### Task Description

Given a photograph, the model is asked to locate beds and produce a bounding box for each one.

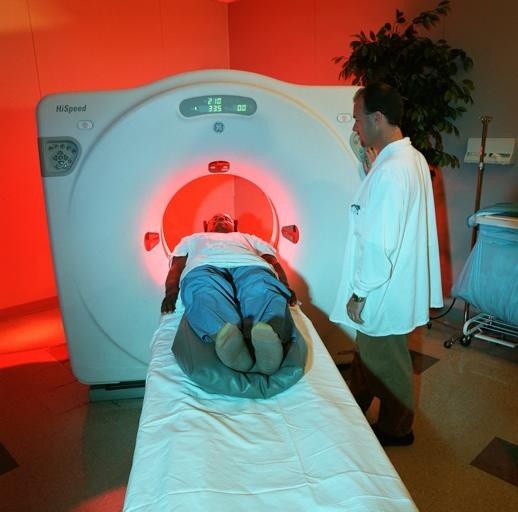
[123,276,417,512]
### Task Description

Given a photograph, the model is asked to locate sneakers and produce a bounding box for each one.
[371,423,414,446]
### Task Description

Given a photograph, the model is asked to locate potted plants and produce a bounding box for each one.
[329,2,477,181]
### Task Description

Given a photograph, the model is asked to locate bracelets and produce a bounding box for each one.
[352,292,366,303]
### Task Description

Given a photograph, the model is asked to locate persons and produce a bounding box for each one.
[328,84,444,447]
[161,212,297,377]
